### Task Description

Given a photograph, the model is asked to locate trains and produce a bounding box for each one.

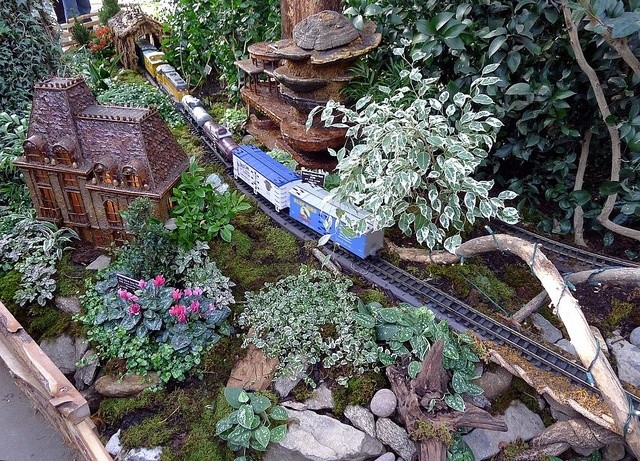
[135,41,384,259]
